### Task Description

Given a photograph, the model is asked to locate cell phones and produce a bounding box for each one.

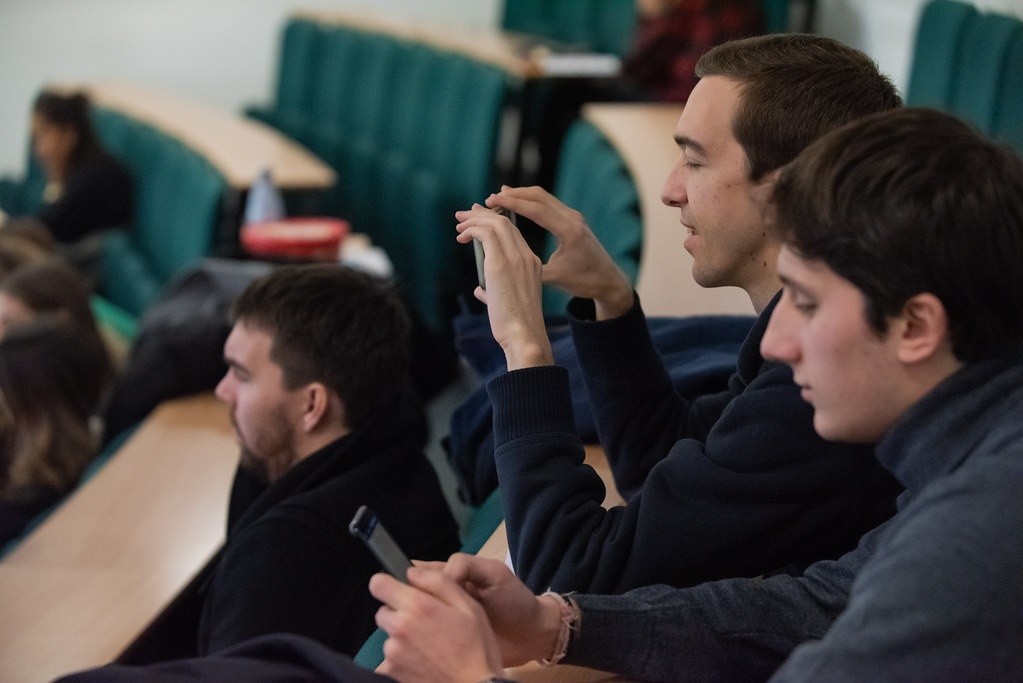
[473,207,516,290]
[349,504,412,586]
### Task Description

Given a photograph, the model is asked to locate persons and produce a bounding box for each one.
[189,260,467,659]
[343,108,1022,682]
[455,32,908,599]
[0,88,133,541]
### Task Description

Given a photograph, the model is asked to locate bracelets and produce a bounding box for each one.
[547,592,579,665]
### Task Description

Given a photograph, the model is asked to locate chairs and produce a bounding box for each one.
[263,3,647,658]
[17,98,234,331]
[904,0,1023,163]
